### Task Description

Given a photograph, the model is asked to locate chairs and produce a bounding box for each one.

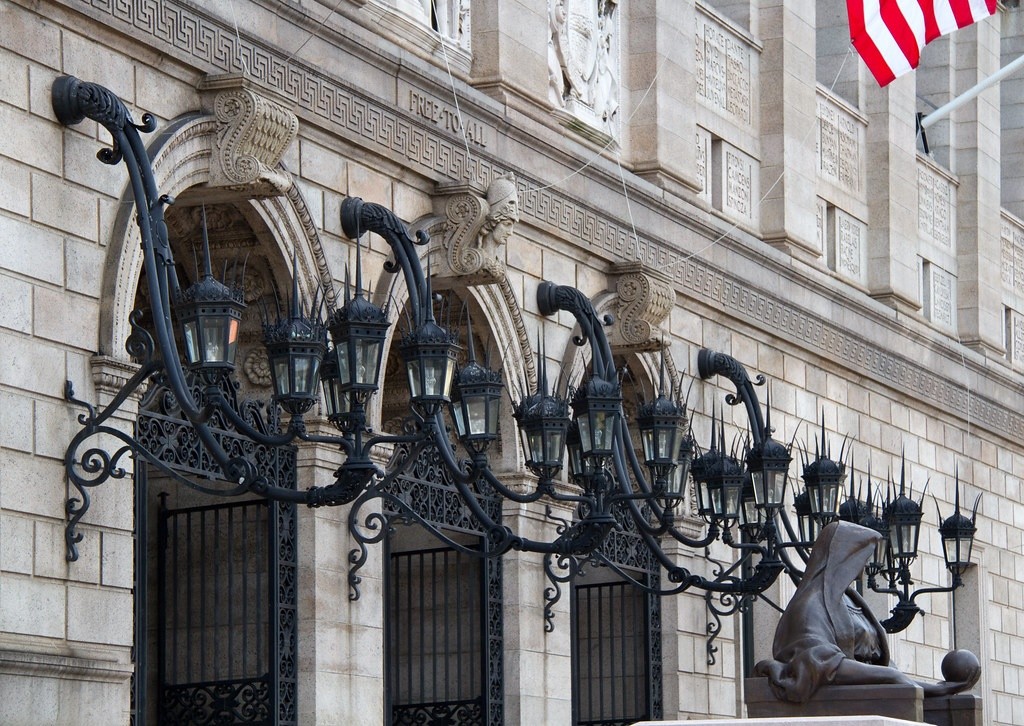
[737,526,984,726]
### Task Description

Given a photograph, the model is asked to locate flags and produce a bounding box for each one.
[846,0,997,87]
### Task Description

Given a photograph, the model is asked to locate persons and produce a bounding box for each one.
[479,173,520,259]
[751,520,982,704]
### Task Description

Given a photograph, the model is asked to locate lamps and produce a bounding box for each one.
[49,74,984,666]
